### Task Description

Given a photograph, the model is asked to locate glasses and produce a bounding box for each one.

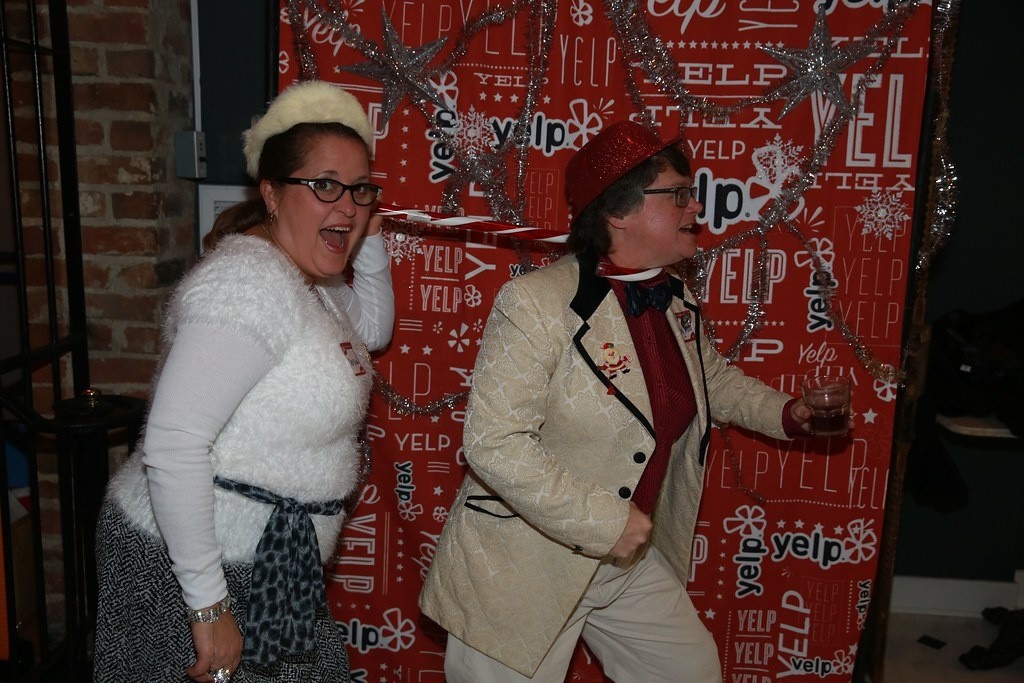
[643,187,698,207]
[278,176,383,206]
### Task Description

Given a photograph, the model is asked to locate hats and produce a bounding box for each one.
[565,120,682,230]
[241,80,372,179]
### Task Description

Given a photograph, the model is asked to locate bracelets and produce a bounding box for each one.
[188,594,231,624]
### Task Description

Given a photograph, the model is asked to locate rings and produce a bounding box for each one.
[209,668,231,683]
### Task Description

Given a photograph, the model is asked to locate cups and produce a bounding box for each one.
[799,375,852,438]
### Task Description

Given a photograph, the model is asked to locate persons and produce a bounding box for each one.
[92,81,397,683]
[418,120,854,683]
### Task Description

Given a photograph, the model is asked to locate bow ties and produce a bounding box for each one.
[624,281,672,318]
